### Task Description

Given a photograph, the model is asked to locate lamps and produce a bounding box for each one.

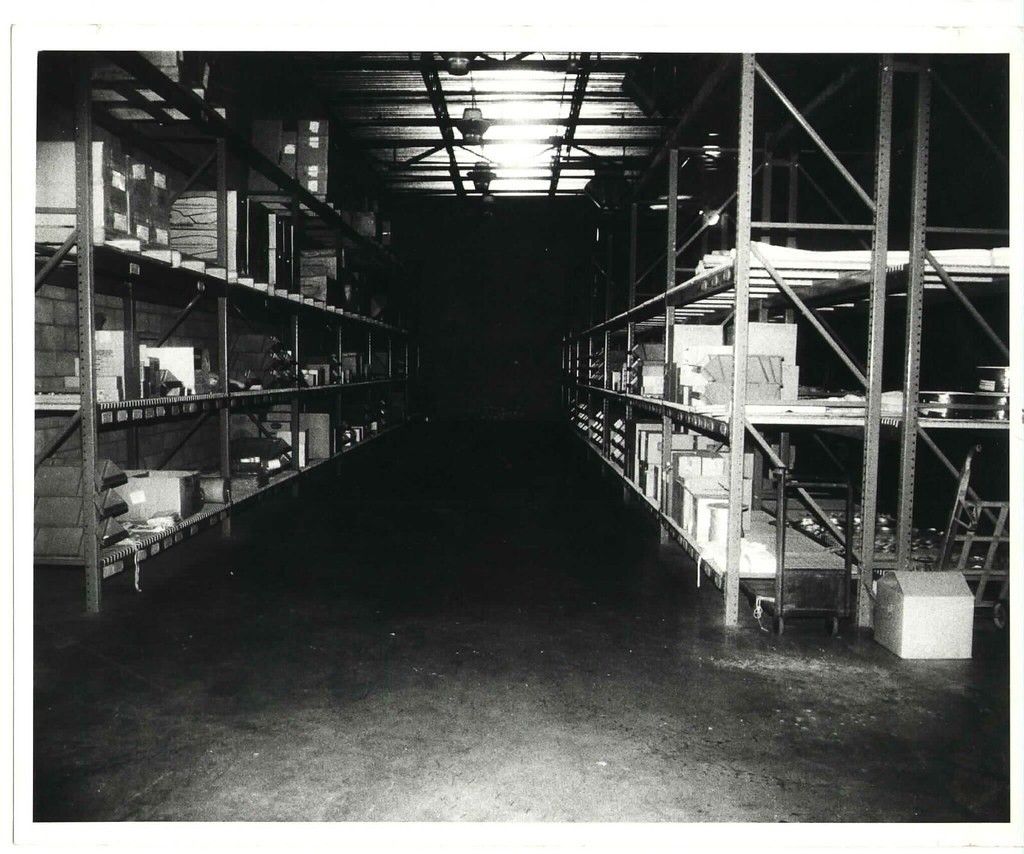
[586,163,633,210]
[438,52,483,75]
[455,92,490,143]
[467,162,496,190]
[622,53,700,116]
[480,195,496,216]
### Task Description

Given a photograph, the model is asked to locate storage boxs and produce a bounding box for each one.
[34,51,406,557]
[570,322,976,659]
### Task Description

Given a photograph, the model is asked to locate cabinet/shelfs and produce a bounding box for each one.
[562,53,1010,626]
[34,51,420,613]
